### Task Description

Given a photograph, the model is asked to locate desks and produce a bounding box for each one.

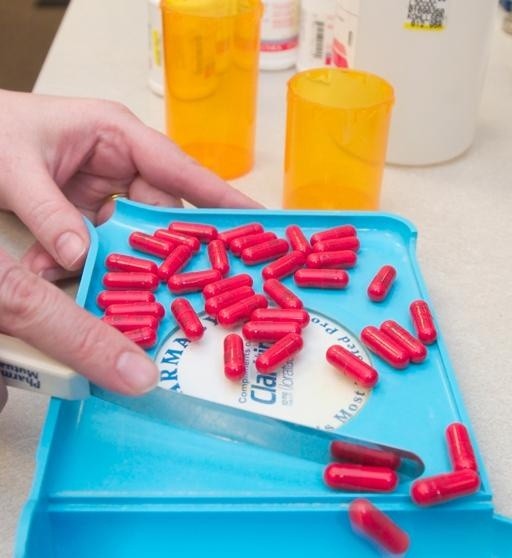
[0,0,512,558]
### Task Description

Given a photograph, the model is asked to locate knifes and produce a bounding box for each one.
[0,326,427,485]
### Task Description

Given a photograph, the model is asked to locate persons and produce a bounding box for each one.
[1,83,270,419]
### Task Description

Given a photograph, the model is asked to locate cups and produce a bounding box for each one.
[158,0,265,185]
[280,64,397,216]
